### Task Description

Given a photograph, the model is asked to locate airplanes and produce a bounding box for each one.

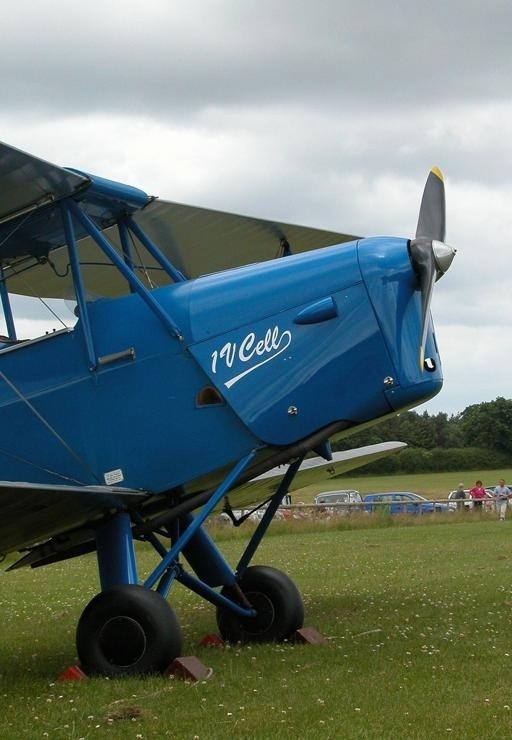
[0,132,461,683]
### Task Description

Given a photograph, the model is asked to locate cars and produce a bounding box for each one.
[217,484,512,524]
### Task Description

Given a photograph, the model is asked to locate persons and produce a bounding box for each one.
[455,483,467,510]
[493,479,510,521]
[469,481,486,510]
